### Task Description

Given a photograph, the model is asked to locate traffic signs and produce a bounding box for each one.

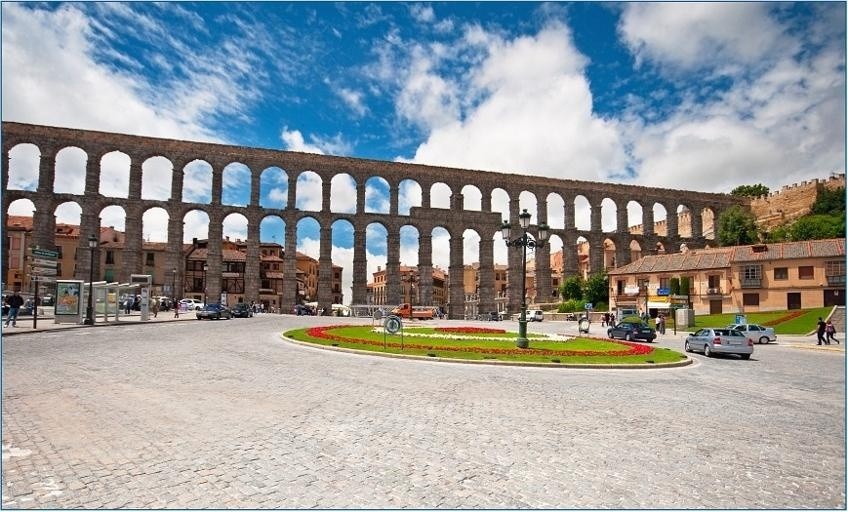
[30,258,57,267]
[31,266,58,275]
[31,276,57,282]
[30,248,59,258]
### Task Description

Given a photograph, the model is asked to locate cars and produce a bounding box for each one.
[1,293,56,307]
[606,320,658,343]
[119,294,206,310]
[230,302,250,317]
[727,323,777,345]
[195,302,231,320]
[684,326,754,361]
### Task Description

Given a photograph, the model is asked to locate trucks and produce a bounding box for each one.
[390,302,434,318]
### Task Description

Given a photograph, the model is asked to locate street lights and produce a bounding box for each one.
[172,267,177,298]
[500,206,553,350]
[401,266,421,323]
[203,262,209,306]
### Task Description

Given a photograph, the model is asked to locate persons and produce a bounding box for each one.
[152,298,159,318]
[601,312,615,328]
[659,314,665,334]
[174,299,180,319]
[825,320,839,345]
[2,292,35,327]
[816,317,827,345]
[655,315,661,331]
[638,308,642,317]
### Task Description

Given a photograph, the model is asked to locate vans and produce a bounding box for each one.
[478,310,498,322]
[518,309,545,323]
